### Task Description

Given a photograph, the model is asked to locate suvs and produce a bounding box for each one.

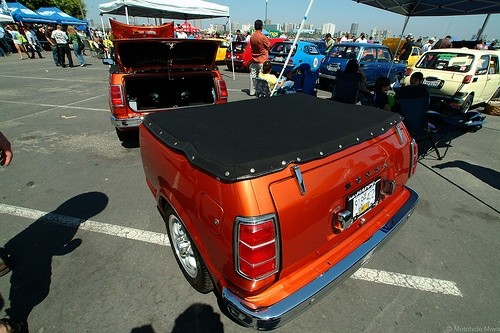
[268,40,329,77]
[315,41,406,85]
[225,39,291,71]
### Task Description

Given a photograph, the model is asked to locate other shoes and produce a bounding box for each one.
[20,57,24,60]
[81,63,85,67]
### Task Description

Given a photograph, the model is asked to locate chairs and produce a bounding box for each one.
[332,69,374,105]
[401,98,480,159]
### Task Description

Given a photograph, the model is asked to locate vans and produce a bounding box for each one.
[403,47,500,114]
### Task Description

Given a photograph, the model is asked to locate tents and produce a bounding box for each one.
[98,0,235,79]
[270,0,500,96]
[174,20,200,32]
[0,1,88,29]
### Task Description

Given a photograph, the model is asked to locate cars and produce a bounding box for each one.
[202,38,246,62]
[102,17,228,147]
[393,45,424,69]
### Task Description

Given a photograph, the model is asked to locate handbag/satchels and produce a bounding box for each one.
[78,38,84,51]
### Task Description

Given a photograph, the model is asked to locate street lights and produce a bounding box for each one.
[265,0,268,21]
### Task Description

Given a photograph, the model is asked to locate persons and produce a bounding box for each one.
[0,131,13,277]
[0,321,28,333]
[394,71,430,109]
[0,22,114,60]
[374,75,390,111]
[395,32,497,63]
[278,32,291,51]
[258,60,294,95]
[45,25,62,66]
[67,26,87,67]
[249,19,271,95]
[316,31,375,55]
[163,23,250,46]
[51,25,77,68]
[343,59,371,106]
